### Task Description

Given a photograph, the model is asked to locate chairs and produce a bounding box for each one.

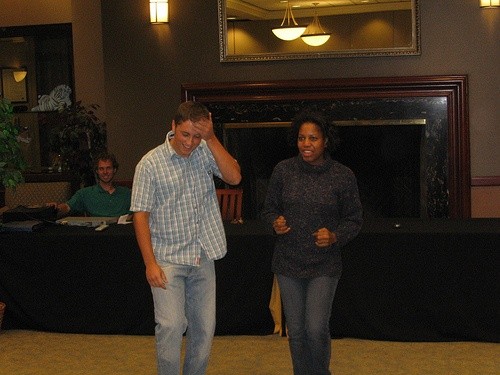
[215,188,243,221]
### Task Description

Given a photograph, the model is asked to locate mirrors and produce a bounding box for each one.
[217,0,422,63]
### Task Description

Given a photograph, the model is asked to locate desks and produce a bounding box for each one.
[0,216,500,345]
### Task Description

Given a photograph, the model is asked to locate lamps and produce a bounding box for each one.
[149,0,170,25]
[300,2,332,47]
[271,1,306,41]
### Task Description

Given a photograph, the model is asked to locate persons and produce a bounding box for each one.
[49,154,132,217]
[262,113,363,375]
[129,100,241,375]
